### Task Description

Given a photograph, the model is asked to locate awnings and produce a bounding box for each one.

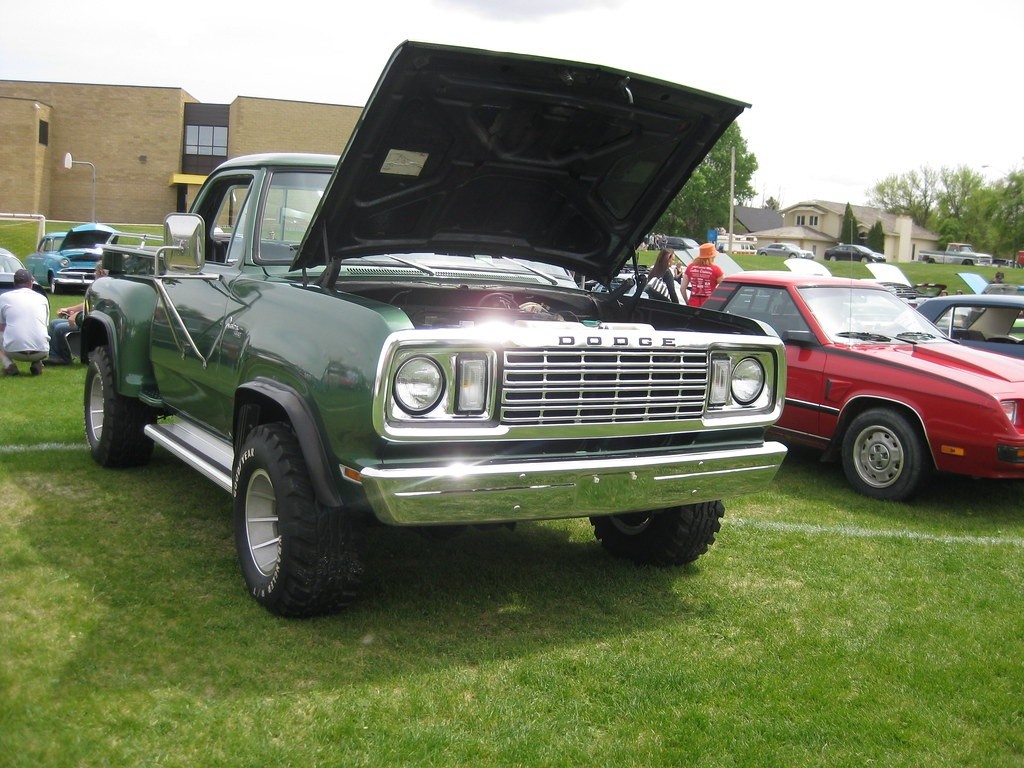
[169,173,208,186]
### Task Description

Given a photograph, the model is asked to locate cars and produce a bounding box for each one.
[756,243,814,262]
[26,222,122,293]
[858,262,1024,360]
[699,271,1024,503]
[0,247,31,290]
[824,244,887,263]
[652,236,744,276]
[78,37,789,625]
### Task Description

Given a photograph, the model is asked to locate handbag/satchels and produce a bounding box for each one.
[644,270,672,303]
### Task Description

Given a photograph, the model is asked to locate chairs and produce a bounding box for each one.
[59,273,128,366]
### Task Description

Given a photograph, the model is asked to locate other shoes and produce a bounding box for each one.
[29,362,42,376]
[42,358,68,367]
[1,364,19,377]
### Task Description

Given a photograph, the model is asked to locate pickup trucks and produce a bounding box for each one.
[918,242,992,267]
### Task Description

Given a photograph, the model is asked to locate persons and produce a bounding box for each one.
[718,225,726,235]
[43,263,108,366]
[640,232,668,251]
[674,261,683,284]
[0,269,49,374]
[680,243,725,308]
[718,244,724,253]
[650,249,679,303]
[989,272,1004,284]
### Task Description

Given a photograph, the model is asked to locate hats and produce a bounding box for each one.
[698,242,719,258]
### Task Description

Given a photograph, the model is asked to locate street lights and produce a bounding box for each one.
[64,152,97,222]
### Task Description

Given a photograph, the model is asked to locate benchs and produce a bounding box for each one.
[214,238,297,263]
[941,328,987,342]
[725,312,801,337]
